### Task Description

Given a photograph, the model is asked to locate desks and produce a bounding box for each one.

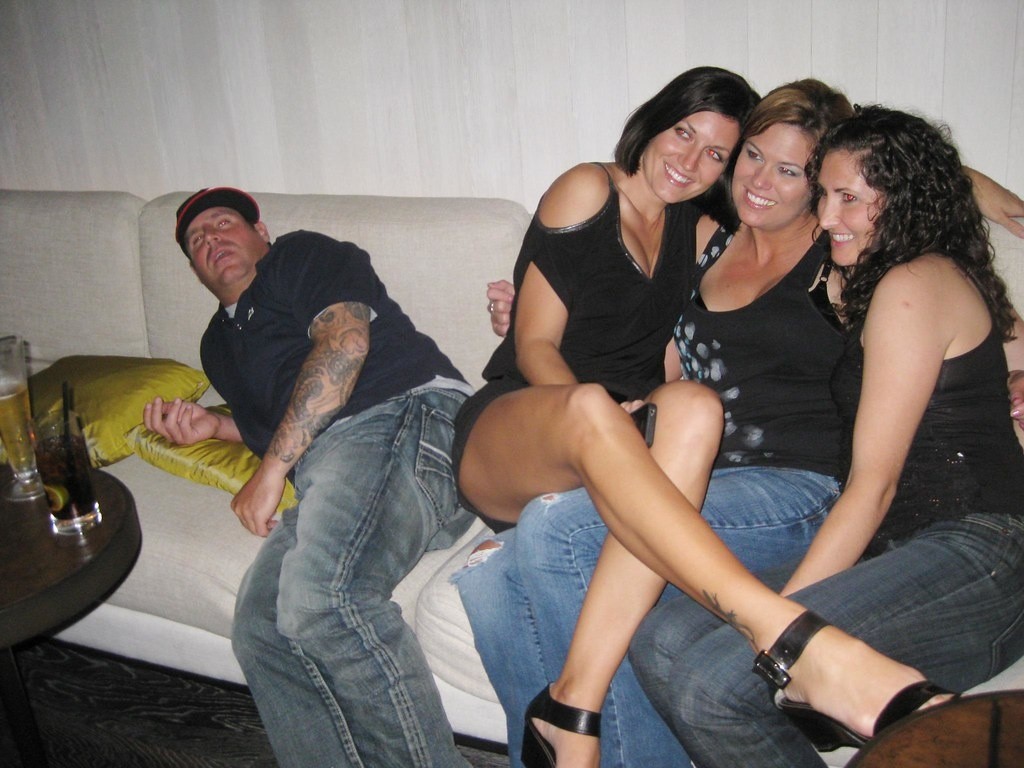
[844,690,1024,767]
[0,464,142,768]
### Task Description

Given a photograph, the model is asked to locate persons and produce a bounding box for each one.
[626,101,1023,768]
[143,187,476,767]
[457,78,1024,768]
[451,67,1024,768]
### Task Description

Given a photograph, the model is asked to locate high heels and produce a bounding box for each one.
[521,681,602,768]
[751,610,959,752]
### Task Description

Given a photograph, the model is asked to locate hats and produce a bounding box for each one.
[175,186,261,261]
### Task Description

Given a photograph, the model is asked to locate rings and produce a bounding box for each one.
[490,301,494,314]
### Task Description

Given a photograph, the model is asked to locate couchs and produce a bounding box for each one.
[0,189,1024,768]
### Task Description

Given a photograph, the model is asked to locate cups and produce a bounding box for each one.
[29,409,102,537]
[0,334,47,503]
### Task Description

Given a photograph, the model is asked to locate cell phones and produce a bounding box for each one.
[630,402,658,448]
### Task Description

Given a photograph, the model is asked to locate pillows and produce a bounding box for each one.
[123,405,298,514]
[29,355,210,469]
